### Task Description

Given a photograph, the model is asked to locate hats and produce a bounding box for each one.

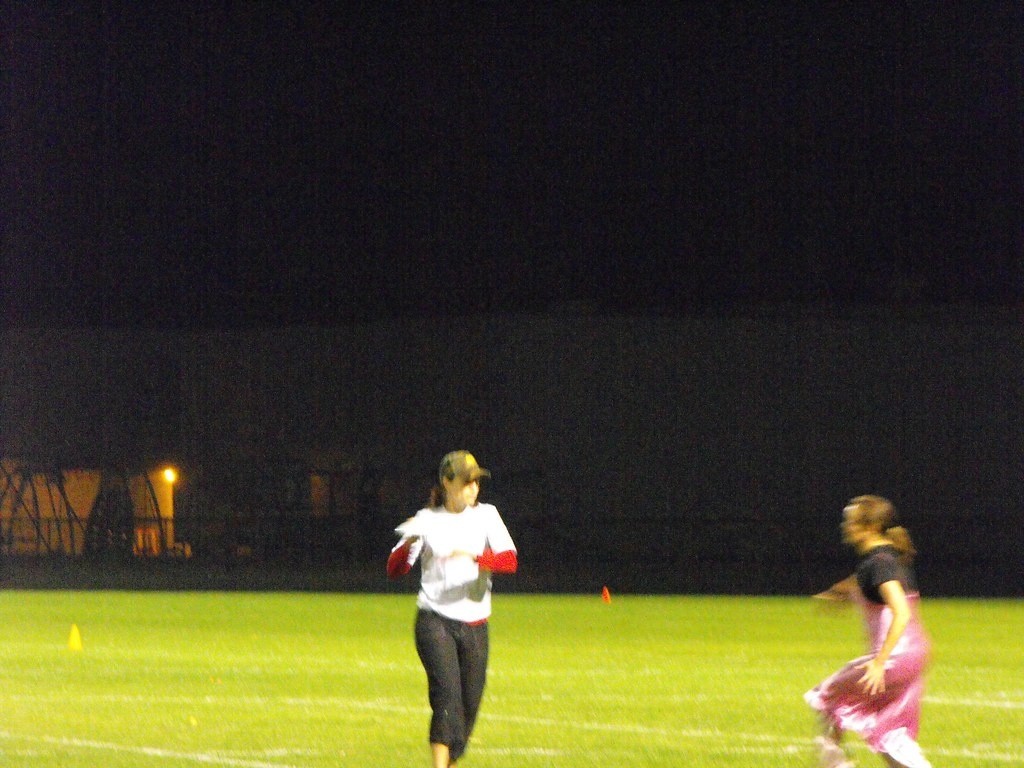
[439,450,492,483]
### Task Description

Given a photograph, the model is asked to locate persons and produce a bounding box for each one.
[806,495,933,768]
[386,451,518,768]
